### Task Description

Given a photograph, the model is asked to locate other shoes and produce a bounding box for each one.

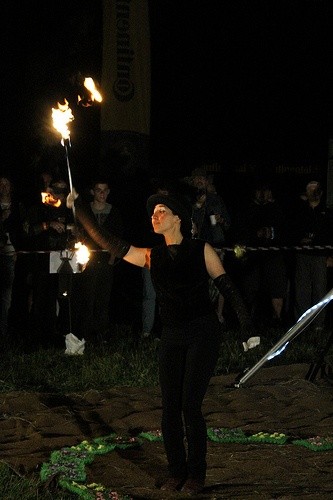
[180,479,204,497]
[160,477,186,492]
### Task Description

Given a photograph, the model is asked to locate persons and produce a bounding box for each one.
[0,167,333,353]
[67,188,261,494]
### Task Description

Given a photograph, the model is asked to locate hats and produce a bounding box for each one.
[145,193,192,222]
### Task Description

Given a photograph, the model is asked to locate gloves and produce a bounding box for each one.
[215,272,261,352]
[66,189,132,260]
